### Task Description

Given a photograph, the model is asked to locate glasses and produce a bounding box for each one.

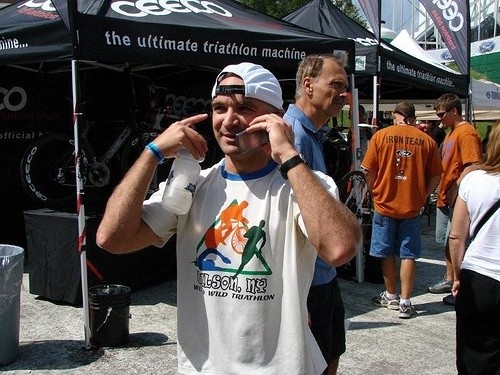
[437,106,455,118]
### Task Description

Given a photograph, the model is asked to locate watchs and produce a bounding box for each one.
[279,154,307,180]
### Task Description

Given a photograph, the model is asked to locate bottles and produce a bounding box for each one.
[161,144,204,215]
[57,168,65,183]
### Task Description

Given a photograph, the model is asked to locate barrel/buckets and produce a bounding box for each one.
[88,284,130,345]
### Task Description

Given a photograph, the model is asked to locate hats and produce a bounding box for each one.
[212,62,284,109]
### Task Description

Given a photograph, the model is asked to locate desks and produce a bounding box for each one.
[25,208,176,307]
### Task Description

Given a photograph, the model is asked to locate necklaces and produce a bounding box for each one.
[448,122,500,375]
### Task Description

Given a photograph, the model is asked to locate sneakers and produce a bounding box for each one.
[399,303,415,318]
[372,291,400,309]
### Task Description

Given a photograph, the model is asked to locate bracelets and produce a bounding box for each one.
[145,141,166,166]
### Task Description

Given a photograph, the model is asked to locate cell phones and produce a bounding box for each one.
[235,129,269,155]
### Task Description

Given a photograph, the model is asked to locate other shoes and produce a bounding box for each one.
[428,275,453,293]
[443,294,455,306]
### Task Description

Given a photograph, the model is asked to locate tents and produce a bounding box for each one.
[278,1,469,96]
[367,29,500,109]
[0,1,357,357]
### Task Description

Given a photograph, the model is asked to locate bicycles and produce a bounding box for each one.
[339,170,374,229]
[19,94,156,201]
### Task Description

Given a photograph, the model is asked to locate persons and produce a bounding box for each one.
[429,95,482,304]
[96,62,362,375]
[345,106,494,147]
[359,102,443,319]
[283,55,348,374]
